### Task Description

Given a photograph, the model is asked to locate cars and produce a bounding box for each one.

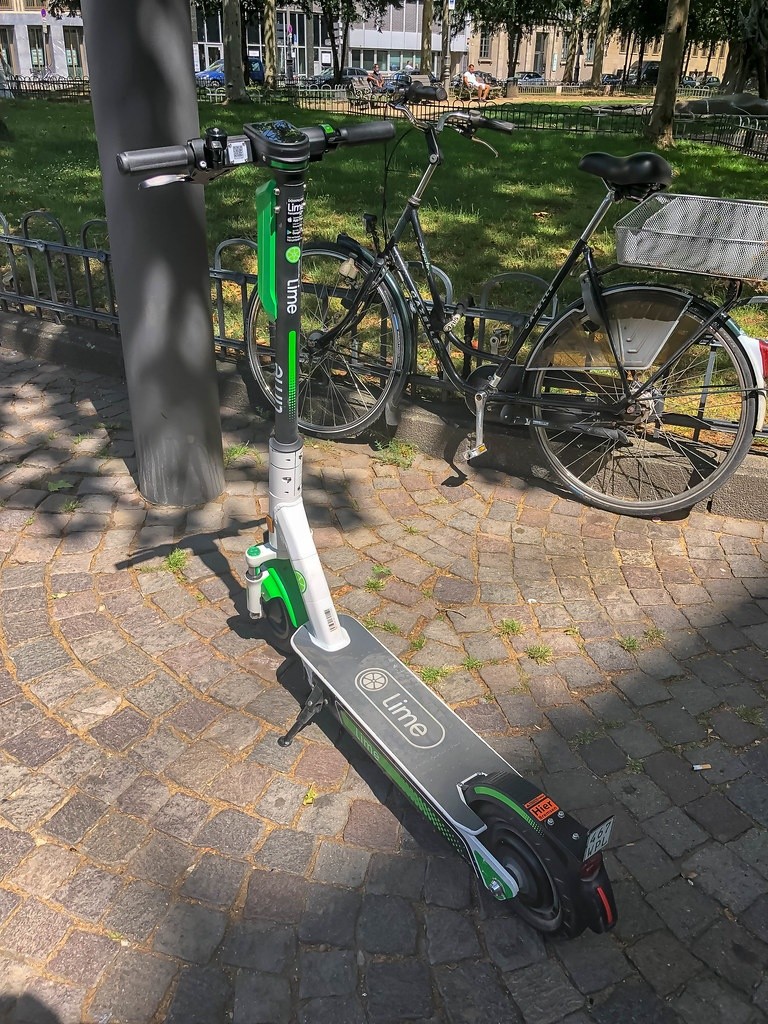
[678,75,696,87]
[696,76,721,87]
[514,72,545,85]
[601,74,622,85]
[307,67,372,86]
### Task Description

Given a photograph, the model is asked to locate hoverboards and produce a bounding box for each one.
[113,120,619,941]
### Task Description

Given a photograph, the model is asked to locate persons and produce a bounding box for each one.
[406,60,413,69]
[464,64,490,102]
[368,64,384,92]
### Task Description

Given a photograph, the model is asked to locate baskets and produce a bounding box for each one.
[615,192,768,280]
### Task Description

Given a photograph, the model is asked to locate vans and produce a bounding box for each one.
[621,60,661,84]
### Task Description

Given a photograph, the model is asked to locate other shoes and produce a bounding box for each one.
[479,98,487,102]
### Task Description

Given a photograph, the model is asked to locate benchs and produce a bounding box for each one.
[396,74,434,105]
[346,74,393,109]
[459,72,504,102]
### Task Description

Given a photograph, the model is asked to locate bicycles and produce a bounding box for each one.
[242,83,768,516]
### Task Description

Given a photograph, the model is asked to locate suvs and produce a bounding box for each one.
[194,56,264,89]
